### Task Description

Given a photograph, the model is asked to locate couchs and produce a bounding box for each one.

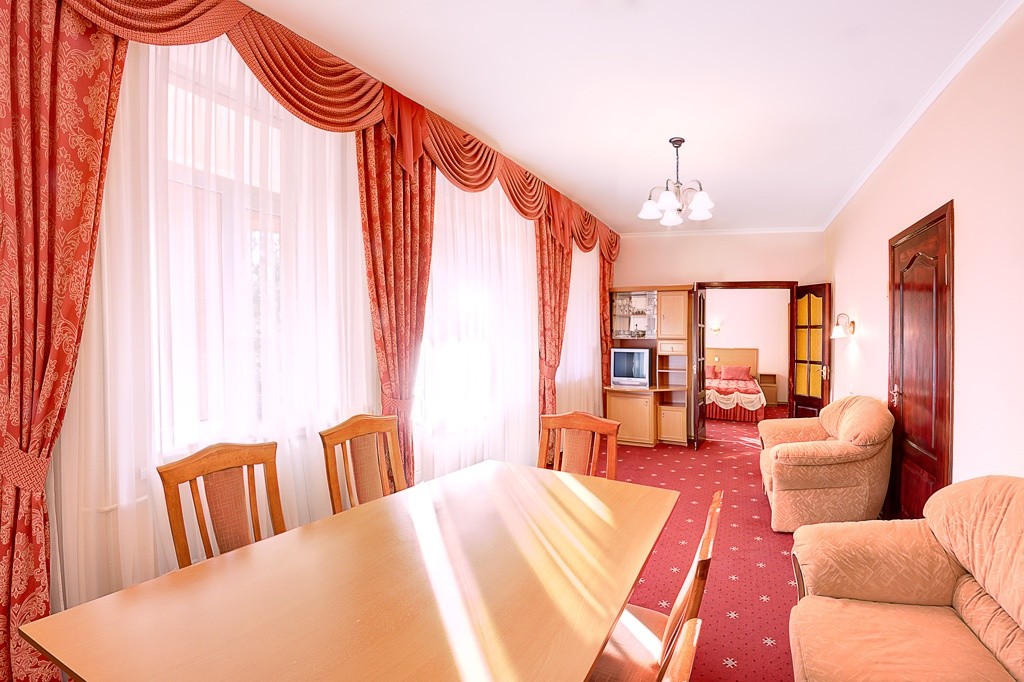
[758,394,895,532]
[789,475,1024,682]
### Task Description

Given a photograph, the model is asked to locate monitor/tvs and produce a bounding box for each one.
[611,348,651,390]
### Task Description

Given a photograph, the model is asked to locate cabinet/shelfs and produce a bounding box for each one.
[608,285,693,448]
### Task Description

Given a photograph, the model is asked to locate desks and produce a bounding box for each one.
[18,459,680,682]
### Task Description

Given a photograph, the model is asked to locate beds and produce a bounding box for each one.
[705,348,767,422]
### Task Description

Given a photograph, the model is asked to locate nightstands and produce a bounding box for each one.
[759,374,778,407]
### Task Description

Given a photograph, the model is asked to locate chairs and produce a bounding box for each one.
[156,442,287,568]
[319,414,407,515]
[537,411,622,480]
[590,490,724,682]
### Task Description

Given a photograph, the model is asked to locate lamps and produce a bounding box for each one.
[829,313,855,339]
[637,137,714,227]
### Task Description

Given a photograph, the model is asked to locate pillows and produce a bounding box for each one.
[706,365,716,378]
[721,365,751,380]
